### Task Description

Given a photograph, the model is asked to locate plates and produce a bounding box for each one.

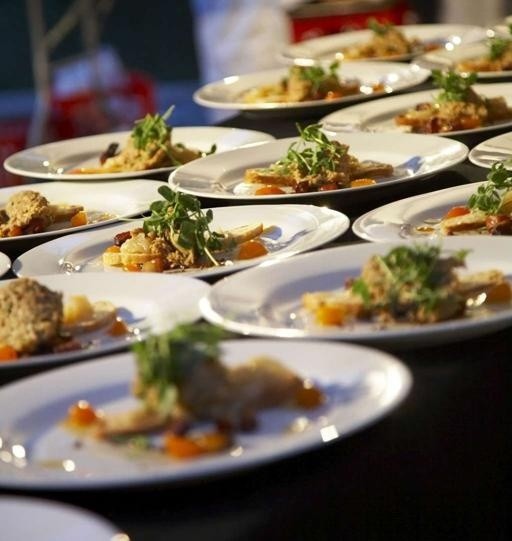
[192,60,431,114]
[320,81,511,135]
[4,125,277,183]
[275,25,496,64]
[199,235,512,341]
[410,38,510,79]
[11,204,351,279]
[0,271,212,367]
[169,132,470,203]
[351,178,512,241]
[1,339,414,491]
[1,177,171,242]
